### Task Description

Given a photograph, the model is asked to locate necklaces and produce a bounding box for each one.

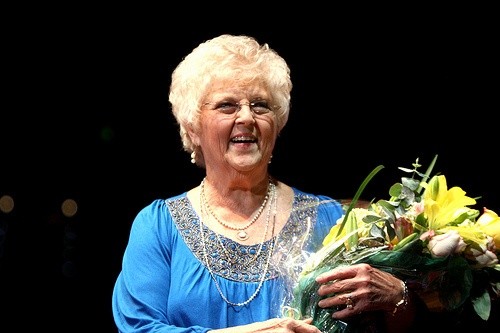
[197,175,277,307]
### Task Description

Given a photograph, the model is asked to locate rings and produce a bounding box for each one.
[344,294,354,310]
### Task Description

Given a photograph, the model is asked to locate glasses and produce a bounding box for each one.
[201,97,281,118]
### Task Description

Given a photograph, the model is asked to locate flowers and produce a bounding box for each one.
[291,155,500,333]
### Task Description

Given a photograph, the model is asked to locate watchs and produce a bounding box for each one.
[395,277,409,310]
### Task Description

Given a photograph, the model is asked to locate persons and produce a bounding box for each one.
[110,34,421,333]
